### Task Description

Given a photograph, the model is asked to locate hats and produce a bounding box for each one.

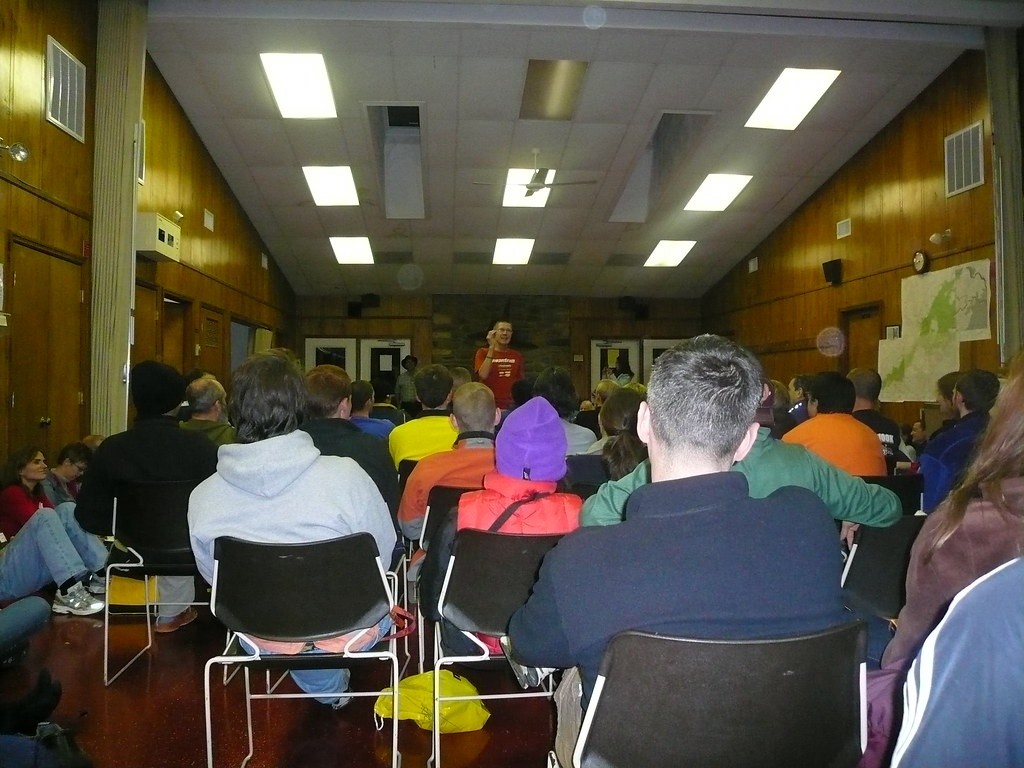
[401,355,418,370]
[131,360,187,415]
[494,398,569,482]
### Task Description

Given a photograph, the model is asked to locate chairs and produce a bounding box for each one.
[104,403,928,768]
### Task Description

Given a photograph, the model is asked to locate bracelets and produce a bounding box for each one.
[487,356,494,360]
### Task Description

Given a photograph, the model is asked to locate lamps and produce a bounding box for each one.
[172,211,184,224]
[930,229,951,245]
[0,137,30,161]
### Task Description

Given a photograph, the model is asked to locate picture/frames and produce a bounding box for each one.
[885,325,900,339]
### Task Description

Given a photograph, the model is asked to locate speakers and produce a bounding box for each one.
[635,305,649,319]
[347,302,362,317]
[822,259,842,282]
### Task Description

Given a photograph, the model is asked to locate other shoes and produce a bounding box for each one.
[154,607,199,632]
[332,687,354,708]
[0,638,29,668]
[501,637,549,691]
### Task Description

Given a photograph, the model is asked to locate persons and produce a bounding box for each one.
[0,347,597,767]
[475,320,525,412]
[858,352,1023,768]
[506,334,854,738]
[571,334,900,580]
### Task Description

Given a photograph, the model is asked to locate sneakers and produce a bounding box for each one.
[88,574,111,593]
[52,582,105,615]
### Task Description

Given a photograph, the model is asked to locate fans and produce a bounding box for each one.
[473,149,598,196]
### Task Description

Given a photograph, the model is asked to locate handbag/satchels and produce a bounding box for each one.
[373,670,490,733]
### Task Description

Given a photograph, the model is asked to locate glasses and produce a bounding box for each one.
[72,461,87,473]
[496,329,513,335]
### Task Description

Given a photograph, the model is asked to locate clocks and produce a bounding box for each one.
[913,250,928,273]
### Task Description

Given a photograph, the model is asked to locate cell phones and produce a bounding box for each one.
[919,408,926,432]
